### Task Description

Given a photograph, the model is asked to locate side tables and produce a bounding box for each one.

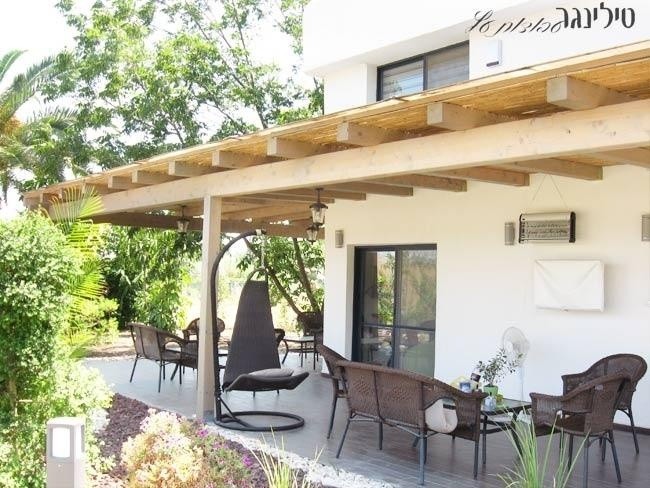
[413,398,532,463]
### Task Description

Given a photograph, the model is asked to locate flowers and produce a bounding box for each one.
[472,341,526,386]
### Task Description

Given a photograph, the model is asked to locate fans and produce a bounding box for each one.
[502,327,530,401]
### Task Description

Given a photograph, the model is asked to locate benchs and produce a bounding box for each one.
[127,320,198,393]
[336,360,489,485]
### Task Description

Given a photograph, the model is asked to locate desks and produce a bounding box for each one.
[360,337,392,361]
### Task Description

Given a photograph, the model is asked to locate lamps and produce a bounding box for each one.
[505,223,514,245]
[642,215,650,241]
[335,230,343,248]
[305,216,324,245]
[309,188,328,231]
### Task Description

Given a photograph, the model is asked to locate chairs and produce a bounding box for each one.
[182,312,323,398]
[316,344,350,439]
[529,369,631,488]
[210,229,309,431]
[224,329,285,364]
[561,354,647,472]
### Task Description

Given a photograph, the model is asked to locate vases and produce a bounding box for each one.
[483,385,498,398]
